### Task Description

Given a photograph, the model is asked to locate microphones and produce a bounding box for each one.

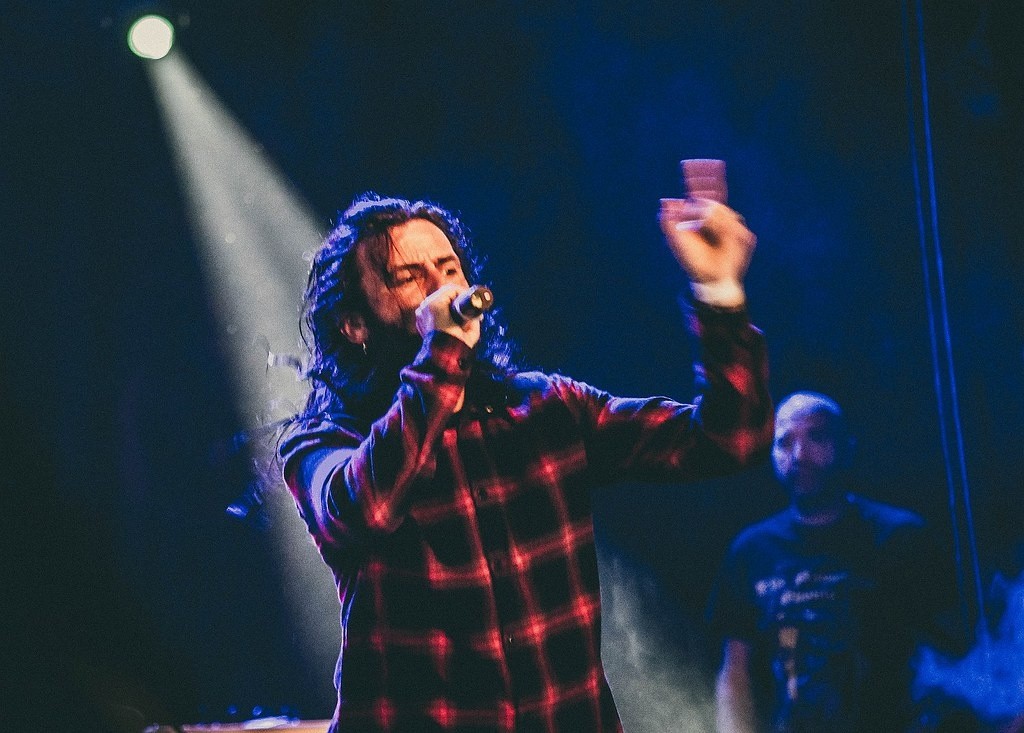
[447,284,493,325]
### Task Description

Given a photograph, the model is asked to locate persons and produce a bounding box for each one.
[714,384,1004,731]
[277,187,781,731]
